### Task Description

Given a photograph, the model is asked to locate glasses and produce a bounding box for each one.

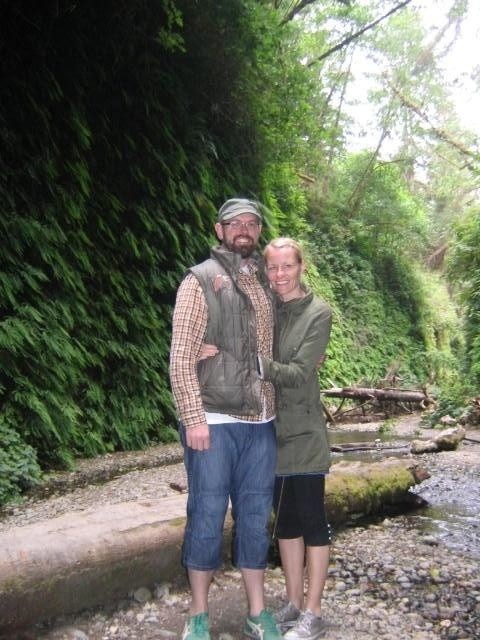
[221,220,259,229]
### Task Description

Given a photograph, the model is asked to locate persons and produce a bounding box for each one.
[199,239,332,640]
[169,198,279,640]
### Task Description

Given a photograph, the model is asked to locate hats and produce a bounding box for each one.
[218,198,262,222]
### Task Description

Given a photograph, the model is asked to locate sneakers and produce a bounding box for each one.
[182,611,211,640]
[244,601,330,640]
